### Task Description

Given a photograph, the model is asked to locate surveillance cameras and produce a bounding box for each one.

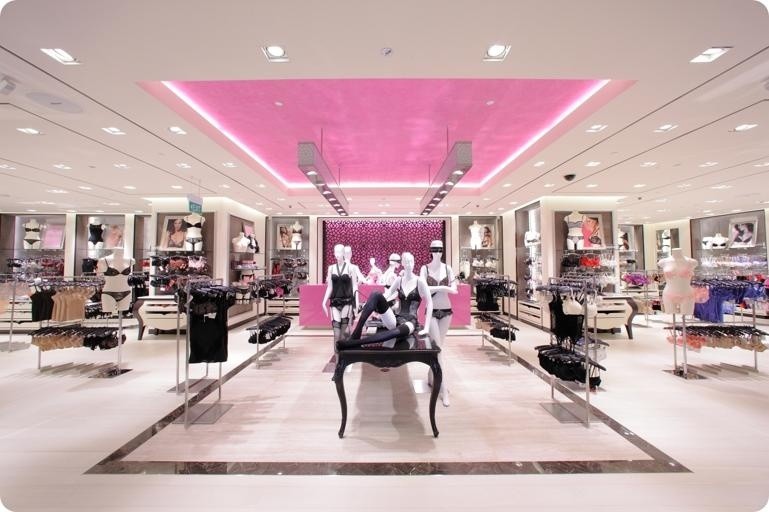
[564,175,576,181]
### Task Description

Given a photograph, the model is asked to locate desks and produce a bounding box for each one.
[331,316,444,442]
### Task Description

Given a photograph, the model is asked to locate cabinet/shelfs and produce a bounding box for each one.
[0,206,769,372]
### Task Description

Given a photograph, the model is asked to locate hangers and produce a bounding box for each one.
[534,333,610,372]
[9,271,105,289]
[535,278,604,295]
[621,268,665,277]
[664,320,768,340]
[691,269,767,289]
[469,271,520,288]
[560,248,613,277]
[6,255,62,265]
[83,301,103,311]
[247,274,293,287]
[174,274,227,293]
[273,253,309,277]
[29,324,119,340]
[474,311,519,333]
[471,254,499,276]
[129,255,207,284]
[245,312,294,331]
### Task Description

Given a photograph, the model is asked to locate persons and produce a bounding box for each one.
[564,211,586,250]
[616,229,629,251]
[163,218,187,248]
[735,223,753,243]
[232,232,251,252]
[732,223,740,241]
[235,260,254,303]
[582,217,599,249]
[523,226,540,245]
[661,230,673,252]
[289,220,303,249]
[183,212,206,251]
[711,233,729,249]
[321,240,457,407]
[279,226,291,248]
[659,248,698,314]
[469,220,483,250]
[482,227,492,247]
[98,247,135,312]
[87,218,105,259]
[247,233,259,253]
[22,218,43,249]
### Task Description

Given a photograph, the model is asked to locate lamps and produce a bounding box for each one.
[295,127,354,220]
[416,124,474,219]
